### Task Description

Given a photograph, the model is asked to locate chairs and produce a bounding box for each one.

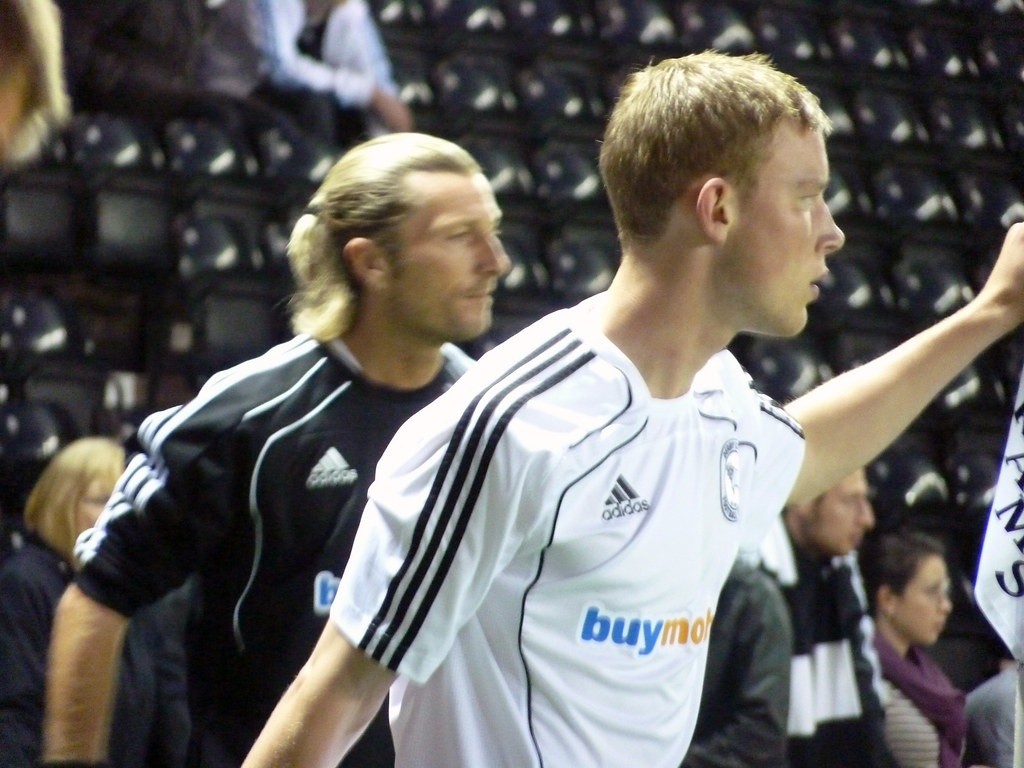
[0,0,1024,560]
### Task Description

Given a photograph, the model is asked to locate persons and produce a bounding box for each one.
[1,1,1023,768]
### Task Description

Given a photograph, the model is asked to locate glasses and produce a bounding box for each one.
[818,484,878,504]
